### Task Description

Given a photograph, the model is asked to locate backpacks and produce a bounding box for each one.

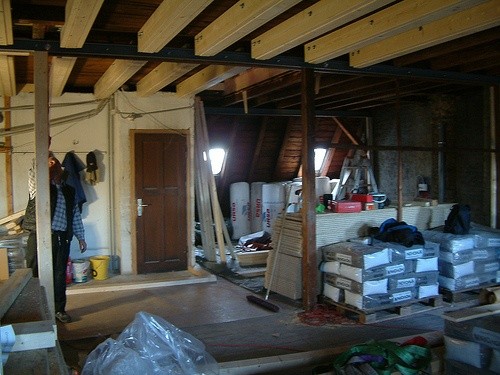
[444,203,472,235]
[369,218,425,247]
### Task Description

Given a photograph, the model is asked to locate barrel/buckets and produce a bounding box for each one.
[66,259,89,284]
[89,255,110,281]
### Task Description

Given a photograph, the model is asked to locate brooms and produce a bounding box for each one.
[246,184,294,311]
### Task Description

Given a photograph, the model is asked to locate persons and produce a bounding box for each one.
[23,158,87,323]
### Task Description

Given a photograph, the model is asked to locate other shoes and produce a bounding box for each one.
[55,312,72,323]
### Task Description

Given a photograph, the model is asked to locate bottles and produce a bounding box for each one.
[0,240,27,275]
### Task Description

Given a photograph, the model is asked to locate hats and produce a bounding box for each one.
[87,152,97,170]
